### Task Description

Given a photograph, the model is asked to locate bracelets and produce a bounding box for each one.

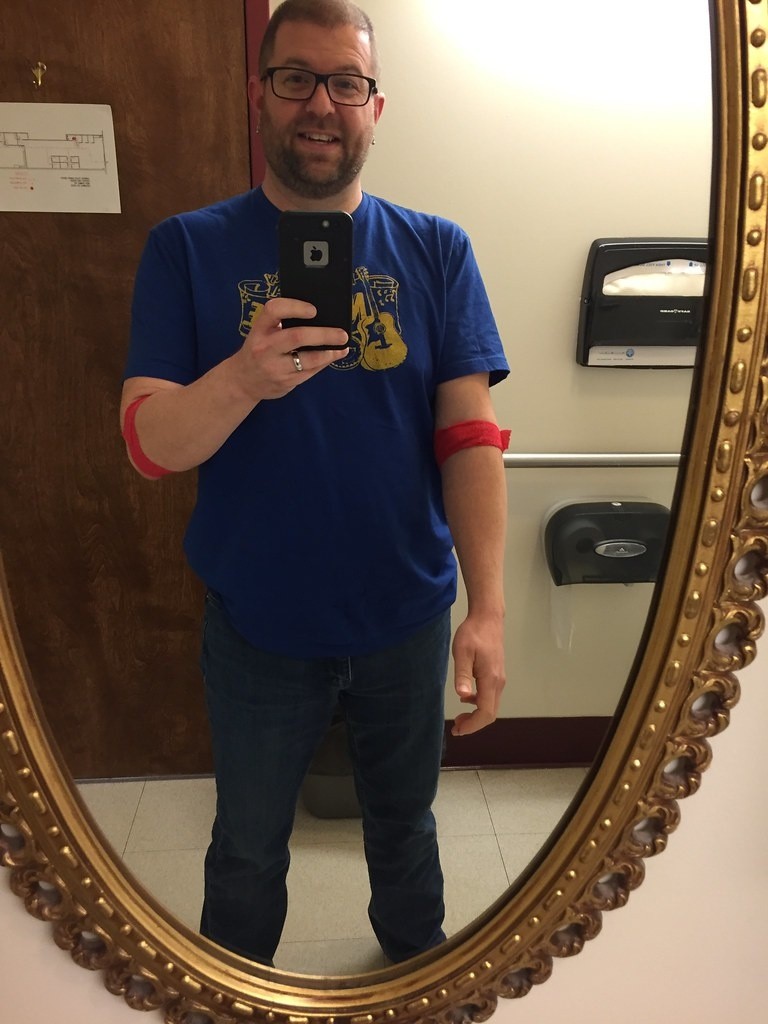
[433,419,512,474]
[124,395,177,478]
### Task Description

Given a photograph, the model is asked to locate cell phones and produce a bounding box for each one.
[279,210,352,351]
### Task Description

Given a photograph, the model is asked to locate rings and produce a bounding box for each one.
[291,351,303,372]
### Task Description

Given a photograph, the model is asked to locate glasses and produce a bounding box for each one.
[260,67,378,108]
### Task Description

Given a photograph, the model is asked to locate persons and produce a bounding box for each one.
[122,0,508,969]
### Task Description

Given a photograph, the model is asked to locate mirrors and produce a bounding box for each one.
[0,0,768,1024]
